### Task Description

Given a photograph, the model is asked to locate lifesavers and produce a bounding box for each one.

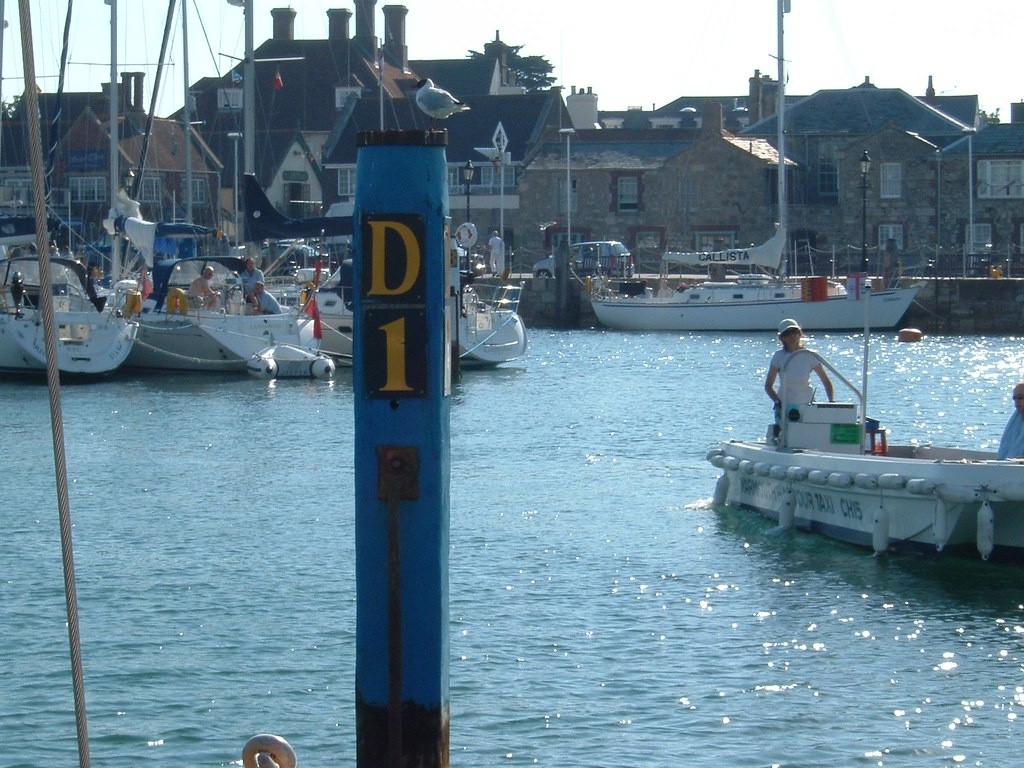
[301,282,318,304]
[167,288,187,315]
[124,292,142,316]
[585,276,591,292]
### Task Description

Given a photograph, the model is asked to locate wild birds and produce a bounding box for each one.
[413,78,476,130]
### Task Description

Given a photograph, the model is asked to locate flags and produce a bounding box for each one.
[272,71,283,91]
[232,70,243,84]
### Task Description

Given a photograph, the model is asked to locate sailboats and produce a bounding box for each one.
[586,0,922,333]
[1,0,528,381]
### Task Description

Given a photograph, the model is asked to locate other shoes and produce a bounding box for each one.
[496,275,499,277]
[493,271,497,277]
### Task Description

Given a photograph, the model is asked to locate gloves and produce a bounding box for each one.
[773,399,782,419]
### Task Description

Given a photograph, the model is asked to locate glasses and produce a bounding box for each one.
[783,329,799,337]
[1012,396,1024,400]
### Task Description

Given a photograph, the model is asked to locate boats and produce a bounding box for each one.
[246,344,336,381]
[706,277,1024,561]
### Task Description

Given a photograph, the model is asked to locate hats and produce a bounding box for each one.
[779,319,800,335]
[203,266,215,273]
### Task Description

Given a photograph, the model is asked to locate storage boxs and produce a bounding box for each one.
[787,421,861,455]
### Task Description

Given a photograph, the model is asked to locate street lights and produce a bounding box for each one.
[228,133,244,247]
[859,150,872,272]
[559,128,574,246]
[962,128,977,276]
[463,160,475,270]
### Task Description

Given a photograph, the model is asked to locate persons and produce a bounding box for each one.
[995,383,1024,461]
[7,242,76,260]
[188,266,220,311]
[249,281,283,315]
[764,317,835,438]
[488,230,502,278]
[657,279,673,297]
[240,257,263,302]
[635,279,653,298]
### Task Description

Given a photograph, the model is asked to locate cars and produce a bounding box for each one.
[533,242,633,278]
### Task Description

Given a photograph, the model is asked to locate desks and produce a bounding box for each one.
[864,428,888,456]
